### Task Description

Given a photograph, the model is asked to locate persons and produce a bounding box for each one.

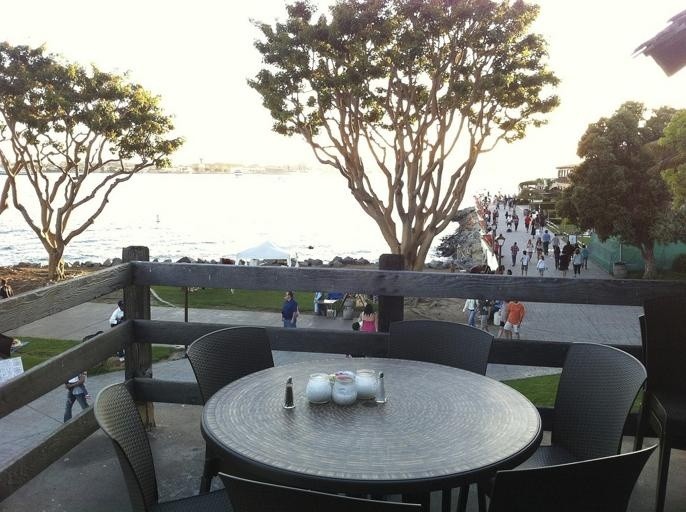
[109,301,126,358]
[462,191,589,340]
[64,371,89,423]
[281,291,299,328]
[0,279,14,299]
[347,322,368,357]
[358,304,378,332]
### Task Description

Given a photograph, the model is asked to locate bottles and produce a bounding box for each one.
[307,370,386,407]
[283,375,296,409]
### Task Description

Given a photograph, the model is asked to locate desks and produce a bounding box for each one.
[201,354,545,511]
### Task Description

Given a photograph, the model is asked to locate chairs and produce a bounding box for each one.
[499,342,649,472]
[386,319,494,376]
[215,471,422,512]
[182,323,275,496]
[487,442,657,511]
[632,293,685,512]
[92,381,275,511]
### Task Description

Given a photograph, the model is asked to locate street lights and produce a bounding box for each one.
[496,234,505,271]
[492,221,497,251]
[485,213,488,233]
